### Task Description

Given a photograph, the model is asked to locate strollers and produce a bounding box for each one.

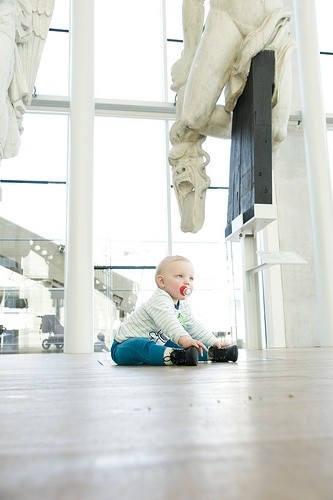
[37,314,111,354]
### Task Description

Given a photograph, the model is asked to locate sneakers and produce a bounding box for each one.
[170,345,198,366]
[208,345,239,361]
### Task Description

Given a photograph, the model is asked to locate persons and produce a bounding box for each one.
[171,0,296,149]
[110,255,238,366]
